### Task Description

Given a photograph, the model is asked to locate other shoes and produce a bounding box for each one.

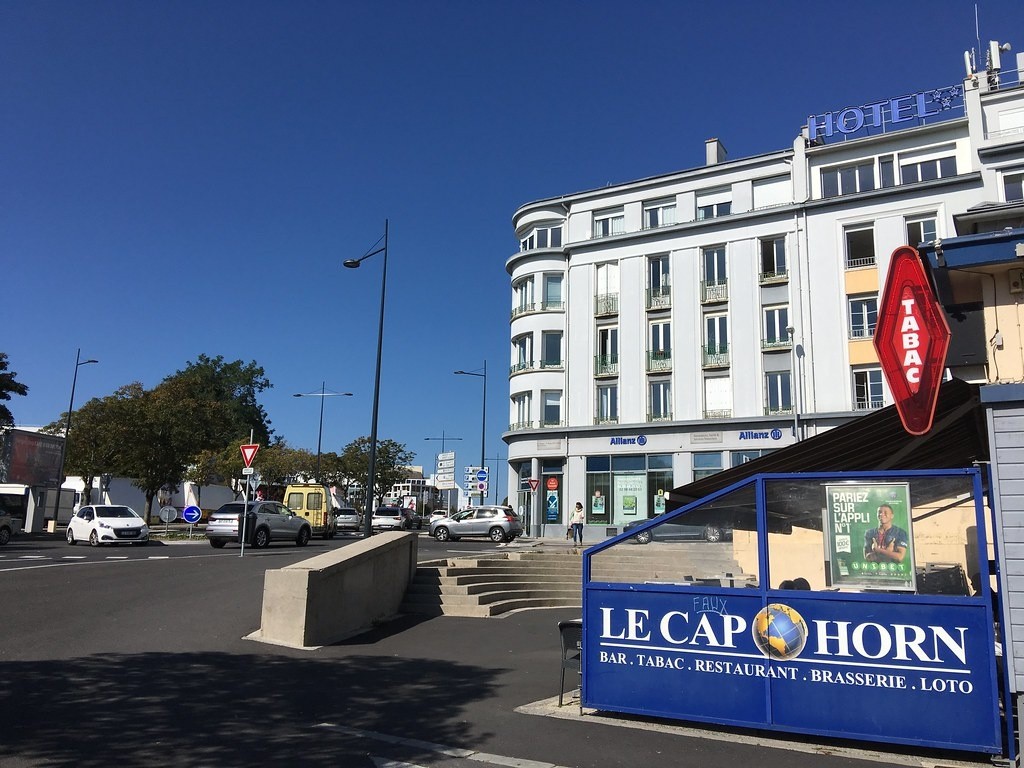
[573,542,577,546]
[579,542,582,546]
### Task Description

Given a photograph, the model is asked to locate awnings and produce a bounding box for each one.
[664,376,994,535]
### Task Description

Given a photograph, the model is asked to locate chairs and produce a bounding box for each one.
[684,575,694,581]
[697,578,721,588]
[557,619,582,707]
[780,578,811,591]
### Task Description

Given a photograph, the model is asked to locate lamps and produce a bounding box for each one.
[934,244,947,267]
[1000,42,1012,52]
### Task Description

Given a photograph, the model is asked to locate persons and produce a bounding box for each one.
[570,502,585,548]
[863,504,907,564]
[408,499,415,509]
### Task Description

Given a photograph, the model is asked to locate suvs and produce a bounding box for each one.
[429,510,452,525]
[335,508,362,532]
[206,501,313,549]
[428,505,523,543]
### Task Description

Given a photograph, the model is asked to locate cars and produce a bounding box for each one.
[371,507,406,531]
[65,504,150,547]
[402,508,422,529]
[623,511,733,545]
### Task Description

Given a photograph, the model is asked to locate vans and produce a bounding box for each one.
[282,482,338,540]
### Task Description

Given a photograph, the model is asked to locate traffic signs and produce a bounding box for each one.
[436,473,455,482]
[435,481,455,490]
[437,459,454,468]
[437,467,455,474]
[437,452,454,461]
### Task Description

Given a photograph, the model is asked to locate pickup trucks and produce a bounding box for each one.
[0,517,22,545]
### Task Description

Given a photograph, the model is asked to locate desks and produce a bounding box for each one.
[695,575,757,588]
[861,588,916,595]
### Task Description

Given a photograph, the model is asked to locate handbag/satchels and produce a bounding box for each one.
[567,527,574,540]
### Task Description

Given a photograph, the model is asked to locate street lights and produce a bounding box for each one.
[343,217,389,538]
[293,381,353,483]
[424,429,463,509]
[454,359,487,505]
[46,348,99,533]
[786,325,798,443]
